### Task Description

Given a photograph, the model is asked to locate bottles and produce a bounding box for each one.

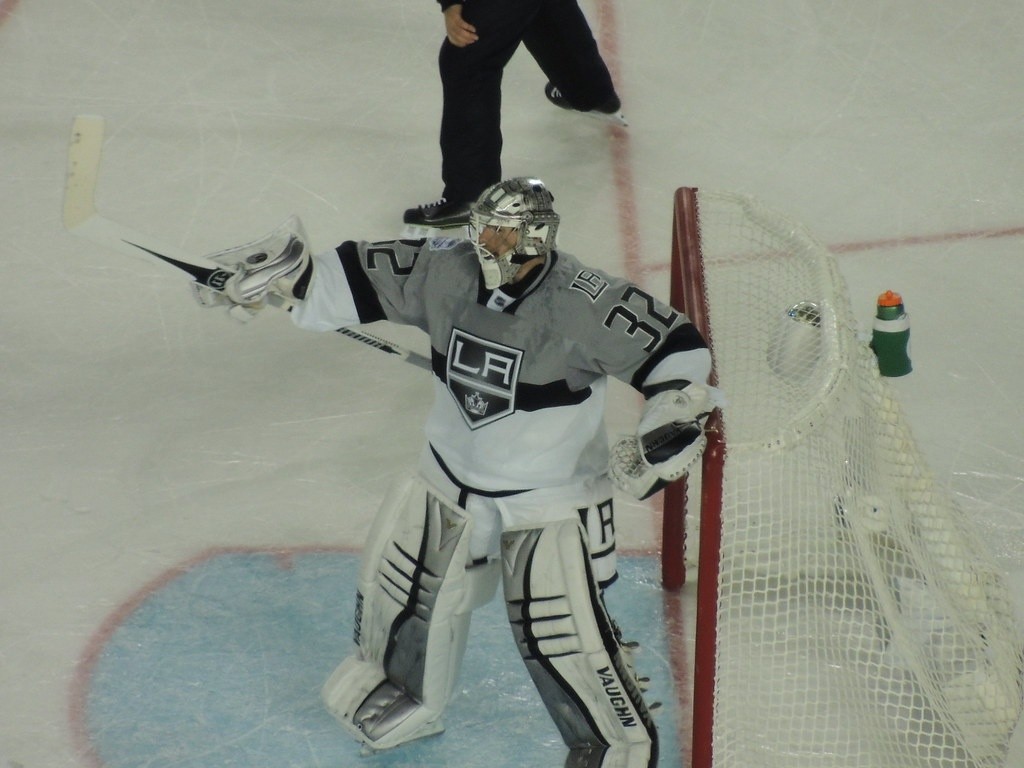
[874,291,912,376]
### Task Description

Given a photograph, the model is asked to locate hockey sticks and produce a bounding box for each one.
[60,112,436,374]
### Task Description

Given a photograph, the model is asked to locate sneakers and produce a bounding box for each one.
[545,80,628,127]
[402,188,473,240]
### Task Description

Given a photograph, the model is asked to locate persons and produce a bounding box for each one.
[190,177,716,768]
[372,1,623,231]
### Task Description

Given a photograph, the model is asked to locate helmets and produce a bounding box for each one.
[467,176,561,290]
